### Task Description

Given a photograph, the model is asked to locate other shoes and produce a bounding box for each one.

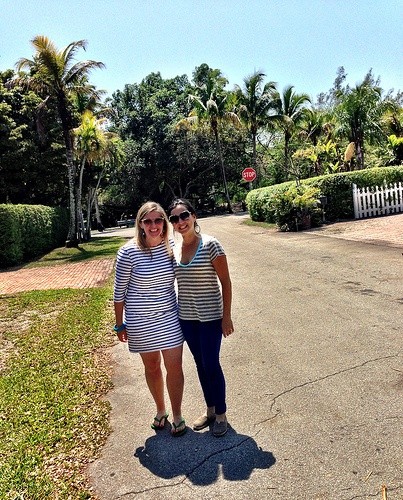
[193,407,227,437]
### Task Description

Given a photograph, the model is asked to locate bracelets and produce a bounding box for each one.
[114,323,127,331]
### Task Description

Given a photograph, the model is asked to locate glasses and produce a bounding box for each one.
[141,217,164,225]
[169,210,191,224]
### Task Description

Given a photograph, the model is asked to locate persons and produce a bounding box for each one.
[166,199,235,437]
[114,202,187,437]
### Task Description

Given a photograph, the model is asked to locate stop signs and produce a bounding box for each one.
[242,168,257,182]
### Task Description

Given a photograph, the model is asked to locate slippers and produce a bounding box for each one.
[171,416,186,437]
[151,413,169,429]
